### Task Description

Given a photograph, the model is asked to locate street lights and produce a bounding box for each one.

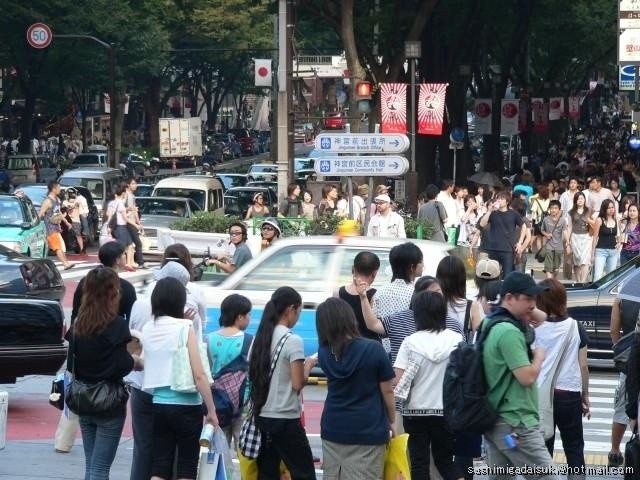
[404,40,423,218]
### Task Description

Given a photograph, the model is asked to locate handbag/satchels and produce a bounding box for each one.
[200,355,251,428]
[238,407,263,460]
[393,339,421,411]
[442,228,448,242]
[47,375,67,412]
[48,212,64,225]
[622,433,639,480]
[534,245,547,264]
[63,374,129,419]
[536,379,556,444]
[313,205,326,222]
[195,437,231,480]
[383,430,412,480]
[237,442,295,479]
[513,242,522,266]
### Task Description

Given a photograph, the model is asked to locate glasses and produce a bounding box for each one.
[375,202,388,206]
[261,226,276,232]
[228,230,244,236]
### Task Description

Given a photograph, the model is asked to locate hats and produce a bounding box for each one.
[374,192,391,203]
[151,260,192,297]
[260,216,282,237]
[375,184,391,196]
[474,258,501,280]
[499,270,550,298]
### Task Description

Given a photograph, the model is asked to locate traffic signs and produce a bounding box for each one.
[27,22,52,49]
[315,133,405,152]
[315,155,406,175]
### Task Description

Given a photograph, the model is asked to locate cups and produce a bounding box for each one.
[494,202,500,212]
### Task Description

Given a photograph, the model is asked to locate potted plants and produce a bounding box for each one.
[158,212,242,257]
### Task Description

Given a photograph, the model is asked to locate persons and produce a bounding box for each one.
[0,81,640,479]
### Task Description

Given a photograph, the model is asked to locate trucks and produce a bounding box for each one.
[157,116,207,166]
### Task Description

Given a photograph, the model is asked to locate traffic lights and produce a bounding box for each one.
[358,81,371,113]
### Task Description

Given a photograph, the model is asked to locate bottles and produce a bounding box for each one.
[199,419,216,446]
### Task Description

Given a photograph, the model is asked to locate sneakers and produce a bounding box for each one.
[607,450,625,469]
[64,262,75,270]
[122,261,150,272]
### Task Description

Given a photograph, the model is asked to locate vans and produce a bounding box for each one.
[57,167,123,240]
[71,153,127,177]
[4,154,62,187]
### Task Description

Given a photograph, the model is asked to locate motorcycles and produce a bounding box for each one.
[138,245,219,292]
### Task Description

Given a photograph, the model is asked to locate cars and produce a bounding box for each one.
[0,292,69,384]
[202,111,349,164]
[0,171,49,258]
[132,146,341,256]
[609,266,640,345]
[12,183,99,250]
[564,253,640,359]
[88,144,108,153]
[1,242,66,303]
[121,152,145,176]
[186,218,483,372]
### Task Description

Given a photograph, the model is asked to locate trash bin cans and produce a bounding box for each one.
[135,161,144,176]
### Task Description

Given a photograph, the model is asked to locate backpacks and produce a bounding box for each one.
[441,315,524,440]
[536,197,550,236]
